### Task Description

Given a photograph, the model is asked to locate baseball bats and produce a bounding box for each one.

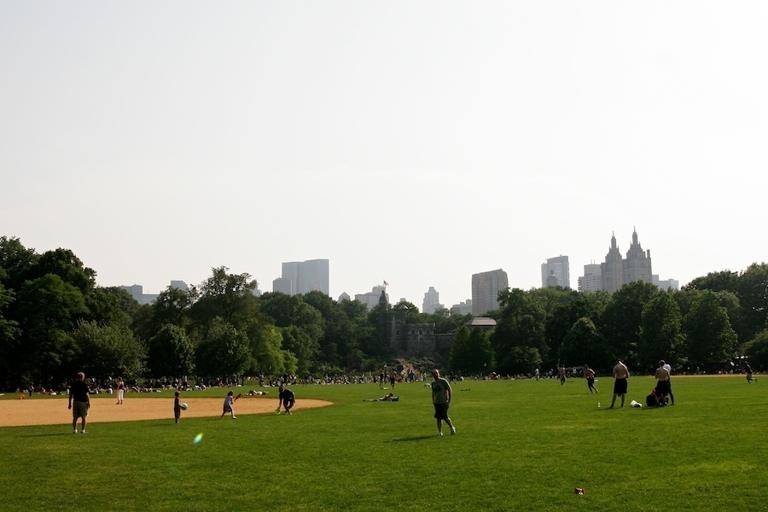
[234,394,241,401]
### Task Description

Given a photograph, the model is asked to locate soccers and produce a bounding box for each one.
[182,403,188,410]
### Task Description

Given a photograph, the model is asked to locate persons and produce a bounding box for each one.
[173,391,185,423]
[221,391,237,419]
[474,365,586,385]
[431,369,456,437]
[279,386,295,415]
[609,359,629,409]
[742,361,757,384]
[646,360,674,407]
[584,364,598,394]
[68,372,90,434]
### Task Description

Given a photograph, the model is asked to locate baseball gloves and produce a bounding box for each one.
[275,408,281,415]
